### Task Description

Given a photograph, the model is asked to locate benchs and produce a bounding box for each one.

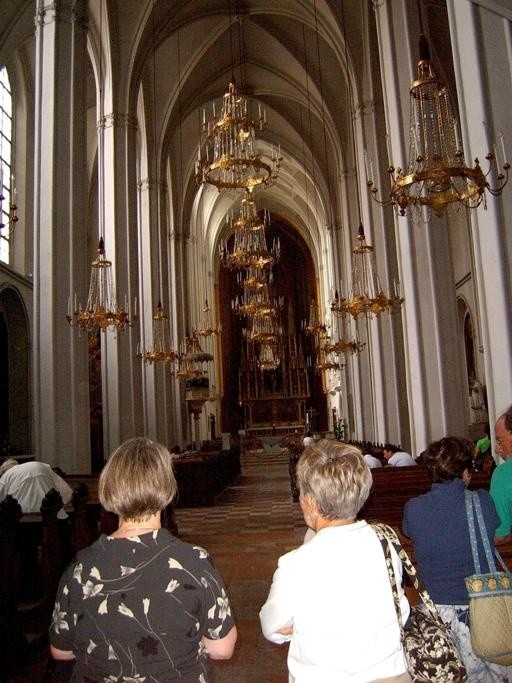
[18,450,241,570]
[288,440,512,605]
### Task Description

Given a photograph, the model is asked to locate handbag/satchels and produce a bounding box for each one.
[400,603,469,683]
[464,570,512,666]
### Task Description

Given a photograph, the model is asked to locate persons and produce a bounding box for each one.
[184,442,200,460]
[363,451,383,467]
[302,430,315,449]
[262,438,430,681]
[168,444,189,459]
[1,453,78,516]
[45,432,241,682]
[390,433,512,683]
[381,443,418,466]
[479,405,512,555]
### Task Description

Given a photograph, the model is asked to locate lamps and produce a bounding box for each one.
[191,0,287,371]
[366,2,511,219]
[296,0,404,372]
[66,0,221,380]
